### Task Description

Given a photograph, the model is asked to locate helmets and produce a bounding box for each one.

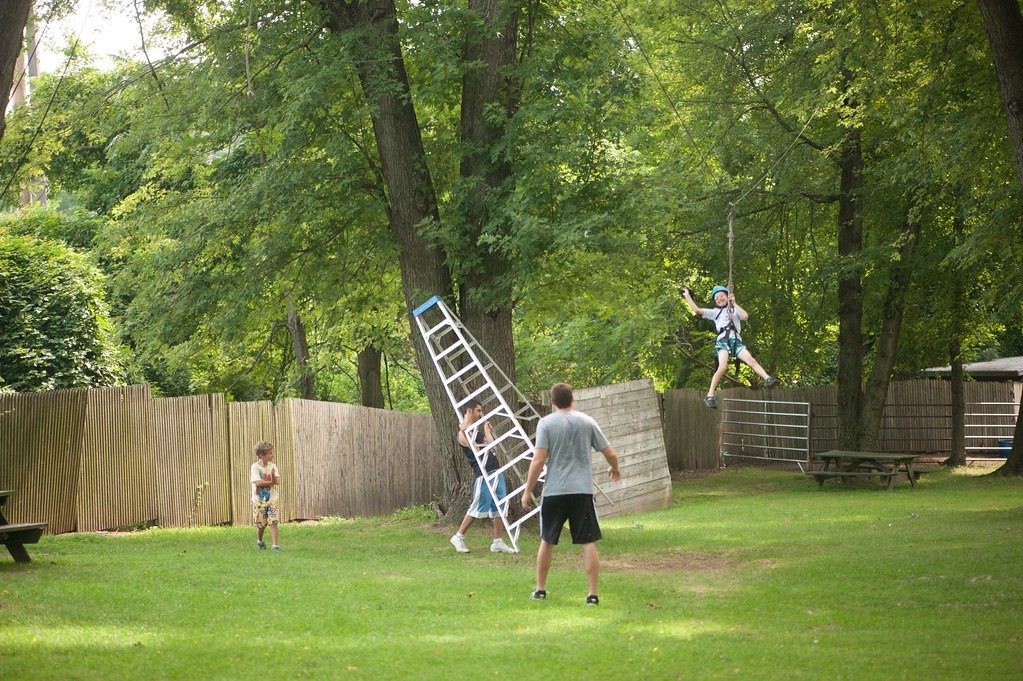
[711,286,728,300]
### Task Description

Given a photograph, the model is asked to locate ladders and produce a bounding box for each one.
[412,295,615,554]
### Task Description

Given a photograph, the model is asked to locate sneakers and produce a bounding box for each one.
[491,540,515,553]
[586,594,599,605]
[449,534,470,553]
[531,590,546,599]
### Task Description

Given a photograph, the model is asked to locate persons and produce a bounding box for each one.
[521,382,620,607]
[680,285,781,408]
[250,441,280,552]
[450,399,517,554]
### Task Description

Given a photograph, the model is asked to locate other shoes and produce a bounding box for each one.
[766,378,780,387]
[704,396,717,409]
[257,539,266,549]
[272,546,280,551]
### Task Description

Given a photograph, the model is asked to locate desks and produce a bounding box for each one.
[815,450,920,491]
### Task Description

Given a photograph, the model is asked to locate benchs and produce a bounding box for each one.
[0,522,49,563]
[843,463,933,473]
[805,471,897,476]
